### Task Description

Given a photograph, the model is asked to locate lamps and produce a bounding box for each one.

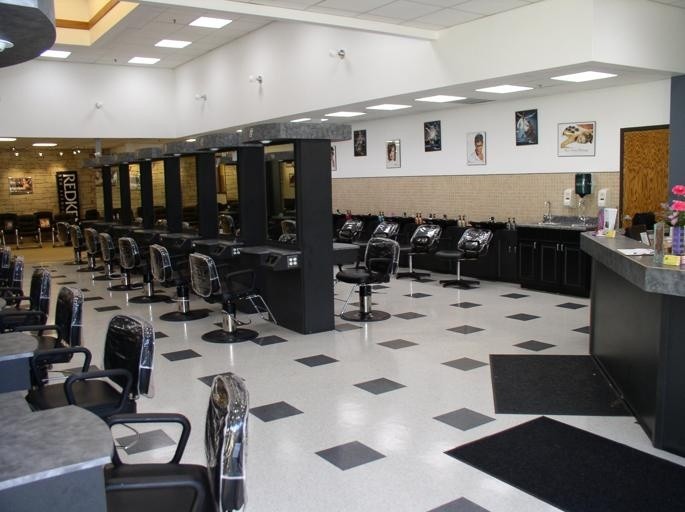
[95,101,102,109]
[249,75,261,80]
[329,49,344,57]
[0,138,16,141]
[33,143,57,147]
[196,92,207,100]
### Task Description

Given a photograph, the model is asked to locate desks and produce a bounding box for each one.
[1,390,111,512]
[0,332,36,394]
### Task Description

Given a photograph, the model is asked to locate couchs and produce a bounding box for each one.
[333,243,358,271]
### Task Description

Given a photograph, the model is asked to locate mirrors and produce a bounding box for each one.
[128,164,144,228]
[150,160,167,228]
[262,144,298,247]
[177,157,200,235]
[110,166,121,224]
[213,151,242,242]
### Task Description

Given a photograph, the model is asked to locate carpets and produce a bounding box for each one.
[489,352,627,416]
[444,417,684,512]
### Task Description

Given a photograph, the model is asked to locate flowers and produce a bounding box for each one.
[655,185,685,227]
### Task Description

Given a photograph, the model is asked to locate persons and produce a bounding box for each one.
[562,124,594,148]
[387,144,396,166]
[467,134,485,163]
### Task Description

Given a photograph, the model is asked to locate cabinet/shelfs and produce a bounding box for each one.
[559,226,591,297]
[580,229,685,455]
[518,224,557,291]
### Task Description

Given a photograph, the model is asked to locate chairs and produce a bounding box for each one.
[64,225,88,265]
[353,222,398,268]
[435,228,492,290]
[189,242,277,344]
[118,237,173,303]
[149,244,208,321]
[335,220,362,243]
[98,232,143,290]
[17,286,91,386]
[0,246,11,286]
[77,228,104,272]
[0,269,50,335]
[24,316,152,428]
[34,211,56,247]
[103,372,250,512]
[0,213,24,247]
[94,233,120,280]
[396,224,442,280]
[0,257,24,310]
[15,214,43,249]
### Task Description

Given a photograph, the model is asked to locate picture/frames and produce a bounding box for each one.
[385,140,402,169]
[330,145,337,172]
[465,131,487,166]
[353,129,367,156]
[515,108,539,145]
[424,119,442,151]
[557,121,597,157]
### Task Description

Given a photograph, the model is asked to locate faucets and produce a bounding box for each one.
[544,201,551,221]
[578,198,584,223]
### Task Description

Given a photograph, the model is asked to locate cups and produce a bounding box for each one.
[604,208,617,232]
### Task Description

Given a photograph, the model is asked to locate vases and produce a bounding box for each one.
[671,227,685,255]
